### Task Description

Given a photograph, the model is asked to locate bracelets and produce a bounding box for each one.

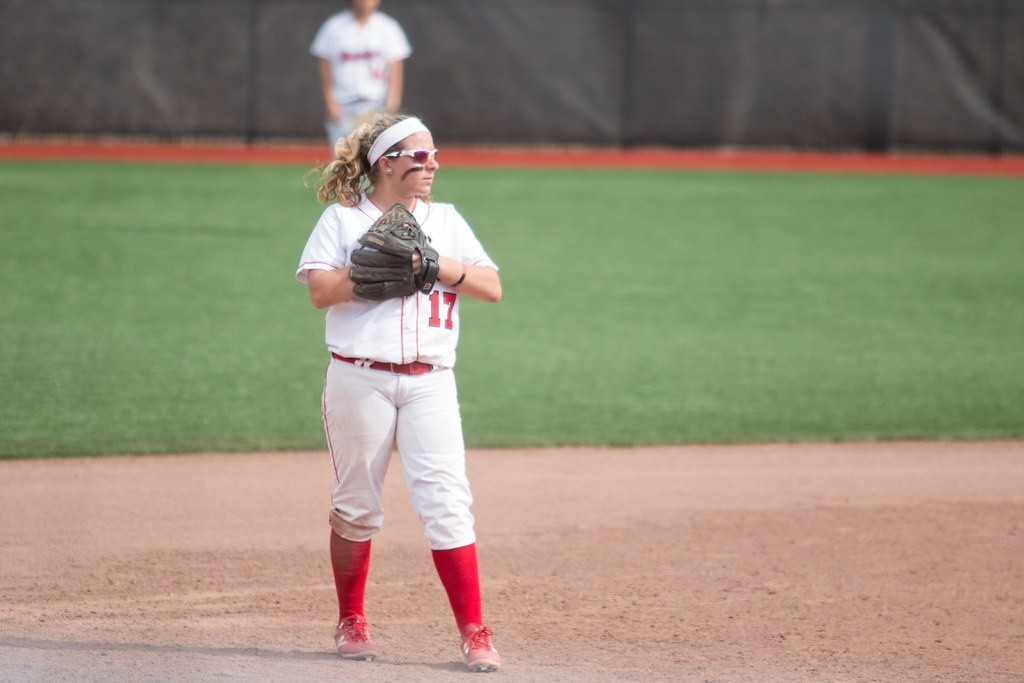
[452,264,467,288]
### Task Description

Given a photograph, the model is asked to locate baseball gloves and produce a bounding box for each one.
[348,202,439,301]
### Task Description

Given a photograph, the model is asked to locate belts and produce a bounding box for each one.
[332,354,433,375]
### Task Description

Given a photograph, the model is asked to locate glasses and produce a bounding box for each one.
[385,149,438,164]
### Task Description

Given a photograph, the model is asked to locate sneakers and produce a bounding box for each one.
[460,624,502,673]
[334,614,378,662]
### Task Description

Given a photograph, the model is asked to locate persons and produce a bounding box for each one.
[294,114,502,672]
[310,0,413,159]
